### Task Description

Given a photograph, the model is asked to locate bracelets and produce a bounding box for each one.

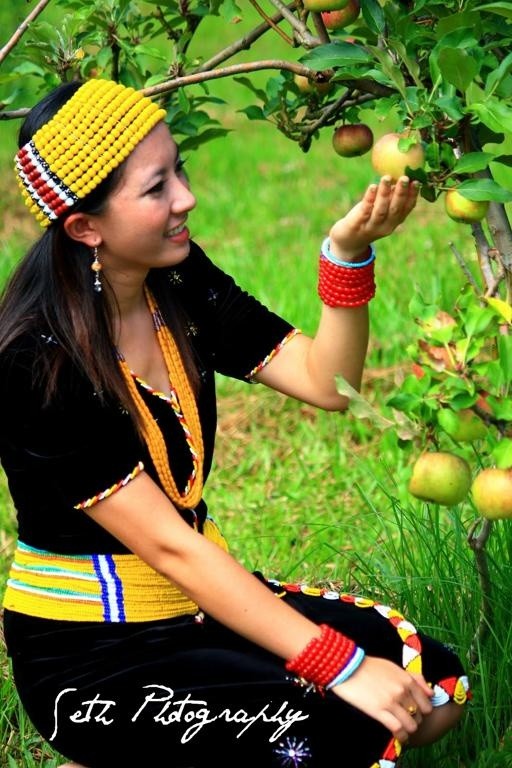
[317,247,377,308]
[320,235,375,268]
[323,645,366,693]
[284,623,356,698]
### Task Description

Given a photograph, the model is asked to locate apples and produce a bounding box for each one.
[299,0,512,519]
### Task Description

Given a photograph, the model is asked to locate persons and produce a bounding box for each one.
[0,79,474,766]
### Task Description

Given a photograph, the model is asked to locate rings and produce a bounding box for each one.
[407,704,419,716]
